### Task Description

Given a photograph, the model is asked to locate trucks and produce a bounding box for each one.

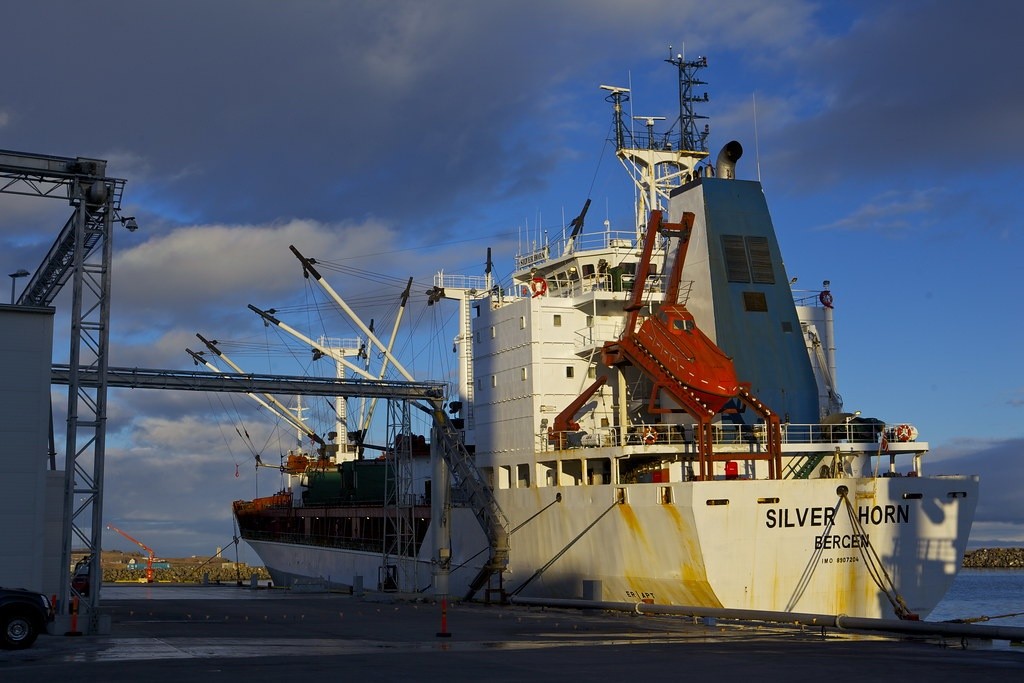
[114,578,148,583]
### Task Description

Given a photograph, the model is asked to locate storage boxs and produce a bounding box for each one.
[652,469,669,483]
[636,472,651,483]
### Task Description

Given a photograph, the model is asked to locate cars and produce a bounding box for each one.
[0,584,56,651]
[142,556,166,564]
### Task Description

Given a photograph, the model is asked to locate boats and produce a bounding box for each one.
[185,36,981,625]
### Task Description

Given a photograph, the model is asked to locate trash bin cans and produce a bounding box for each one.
[378,564,398,592]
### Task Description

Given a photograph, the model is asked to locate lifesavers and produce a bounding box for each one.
[531,277,547,294]
[908,426,919,441]
[897,424,912,441]
[643,427,659,444]
[882,439,890,453]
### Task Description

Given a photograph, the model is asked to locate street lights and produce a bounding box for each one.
[80,496,87,551]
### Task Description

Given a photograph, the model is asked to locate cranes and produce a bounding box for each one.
[106,523,155,582]
